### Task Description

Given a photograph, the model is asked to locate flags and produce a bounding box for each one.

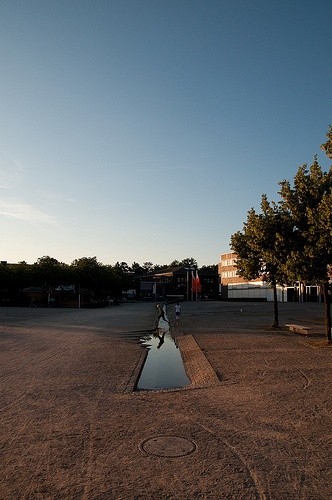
[191,271,197,292]
[153,282,156,294]
[196,270,202,293]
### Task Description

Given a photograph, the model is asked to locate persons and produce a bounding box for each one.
[174,302,181,316]
[155,304,170,328]
